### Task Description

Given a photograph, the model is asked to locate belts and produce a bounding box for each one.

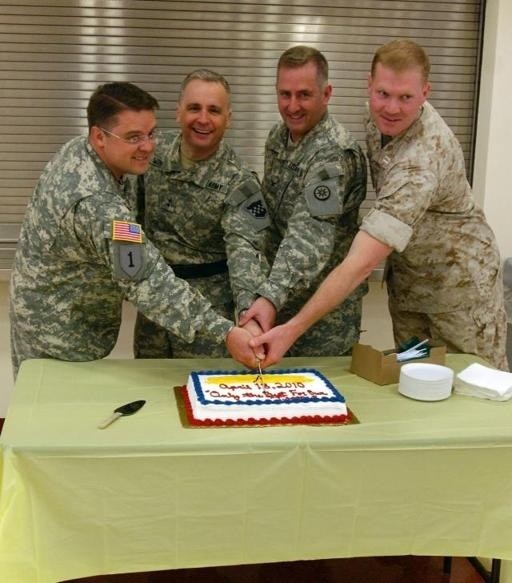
[168,258,227,278]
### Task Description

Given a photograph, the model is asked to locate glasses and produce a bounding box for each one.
[101,127,160,143]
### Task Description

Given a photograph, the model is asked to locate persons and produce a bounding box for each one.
[128,68,264,369]
[253,38,509,382]
[241,45,369,356]
[8,80,264,381]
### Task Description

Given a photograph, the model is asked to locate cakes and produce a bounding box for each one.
[181,369,352,426]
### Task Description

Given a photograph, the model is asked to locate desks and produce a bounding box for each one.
[3,355,509,581]
